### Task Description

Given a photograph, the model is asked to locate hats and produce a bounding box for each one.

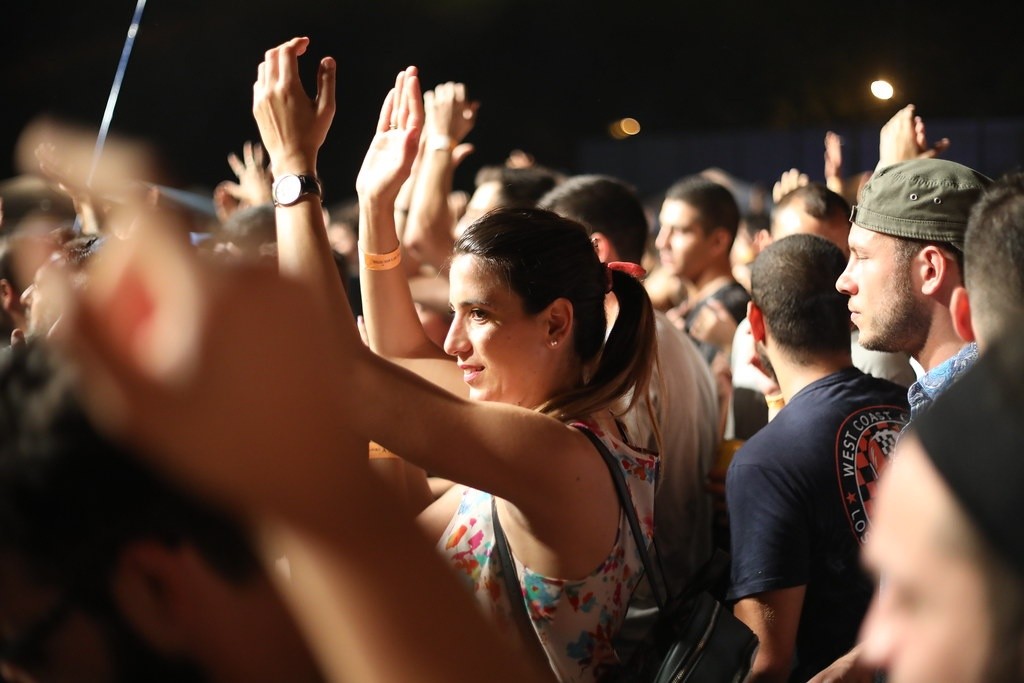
[849,159,996,254]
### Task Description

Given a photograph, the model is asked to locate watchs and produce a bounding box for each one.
[271,174,321,206]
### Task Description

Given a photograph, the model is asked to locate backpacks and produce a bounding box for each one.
[492,423,760,683]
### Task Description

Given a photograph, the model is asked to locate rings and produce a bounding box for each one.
[388,124,398,129]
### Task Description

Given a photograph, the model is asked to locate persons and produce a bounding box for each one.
[0,37,1024,683]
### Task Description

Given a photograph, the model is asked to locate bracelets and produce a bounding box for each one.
[424,134,457,153]
[358,240,402,271]
